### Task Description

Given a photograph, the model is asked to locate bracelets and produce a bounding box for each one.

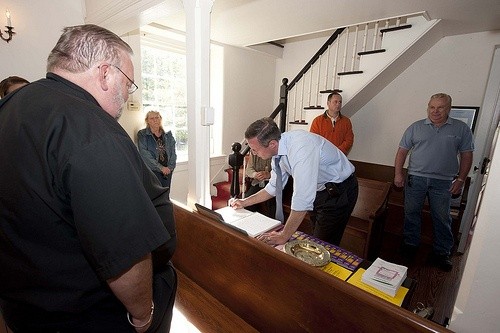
[127,300,155,329]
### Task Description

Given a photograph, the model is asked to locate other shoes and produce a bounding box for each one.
[398,247,421,273]
[433,250,452,272]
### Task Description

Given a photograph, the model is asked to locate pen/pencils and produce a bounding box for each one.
[229,195,237,207]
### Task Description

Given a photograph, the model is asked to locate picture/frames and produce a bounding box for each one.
[448,106,481,134]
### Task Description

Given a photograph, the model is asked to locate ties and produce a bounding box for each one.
[275,155,284,223]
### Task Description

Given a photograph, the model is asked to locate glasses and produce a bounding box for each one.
[98,64,138,94]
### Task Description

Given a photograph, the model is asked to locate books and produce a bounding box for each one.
[361,256,409,298]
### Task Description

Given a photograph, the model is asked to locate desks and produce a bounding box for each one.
[282,176,394,259]
[192,201,418,311]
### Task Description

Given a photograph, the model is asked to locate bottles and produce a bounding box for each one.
[259,180,264,187]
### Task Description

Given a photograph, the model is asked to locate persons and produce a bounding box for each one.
[245,151,284,218]
[392,93,474,271]
[0,76,30,99]
[0,25,171,332]
[228,116,361,247]
[135,109,178,195]
[309,91,355,160]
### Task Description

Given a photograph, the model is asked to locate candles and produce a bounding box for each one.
[7,7,12,27]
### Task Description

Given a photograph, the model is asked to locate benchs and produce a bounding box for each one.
[346,157,472,257]
[169,197,457,333]
